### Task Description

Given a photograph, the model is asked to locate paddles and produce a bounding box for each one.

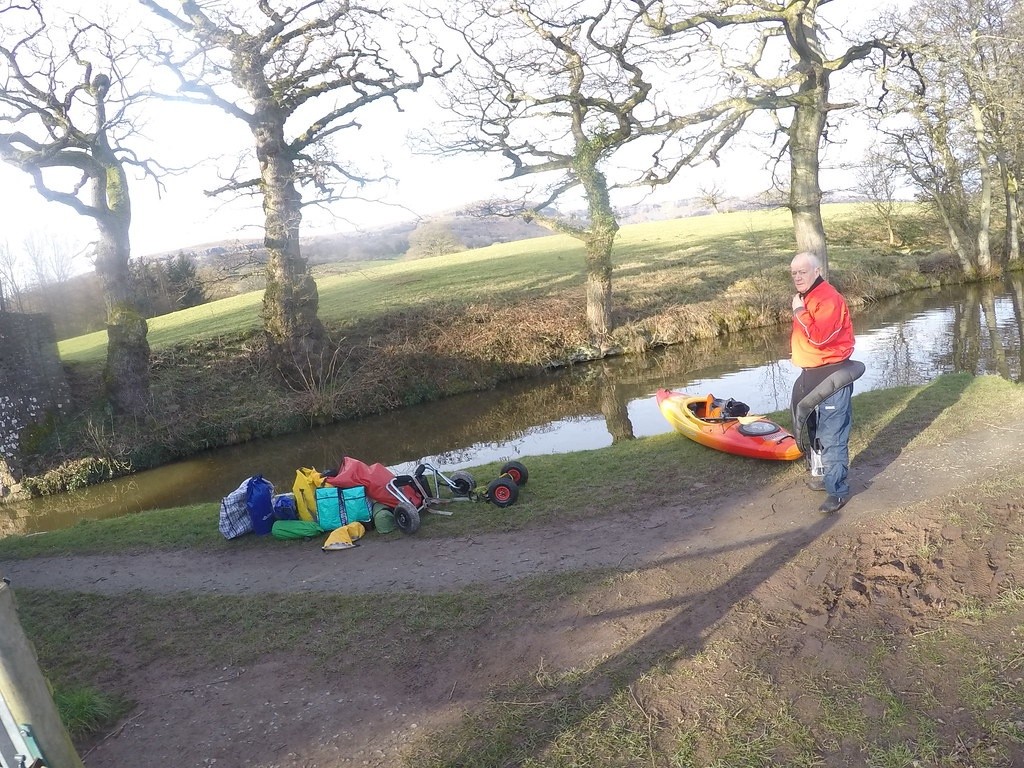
[698,415,767,425]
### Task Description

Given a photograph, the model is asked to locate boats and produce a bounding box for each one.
[653,386,805,461]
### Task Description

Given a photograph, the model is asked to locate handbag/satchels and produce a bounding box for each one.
[219,457,422,550]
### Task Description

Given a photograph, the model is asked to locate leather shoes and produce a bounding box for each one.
[818,495,847,513]
[808,481,825,491]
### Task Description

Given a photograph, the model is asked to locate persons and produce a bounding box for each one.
[790,253,855,512]
